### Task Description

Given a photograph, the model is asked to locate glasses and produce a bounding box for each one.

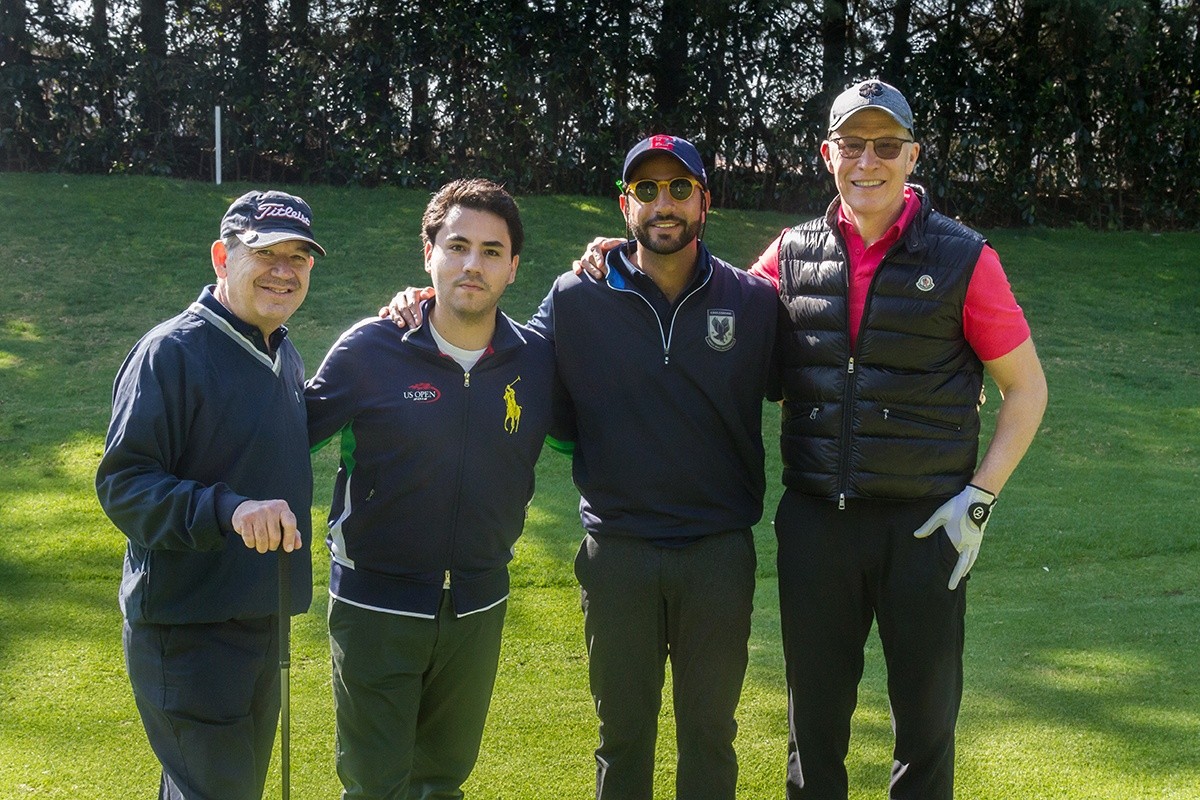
[625,177,704,204]
[829,137,913,159]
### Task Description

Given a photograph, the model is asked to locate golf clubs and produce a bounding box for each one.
[277,525,290,800]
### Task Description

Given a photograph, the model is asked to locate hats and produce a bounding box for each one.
[220,190,326,256]
[827,80,914,138]
[622,134,707,185]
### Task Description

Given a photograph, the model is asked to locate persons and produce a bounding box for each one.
[573,81,1048,800]
[378,135,793,800]
[302,179,570,800]
[95,189,326,800]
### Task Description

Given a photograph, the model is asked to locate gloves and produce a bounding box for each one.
[913,484,998,590]
